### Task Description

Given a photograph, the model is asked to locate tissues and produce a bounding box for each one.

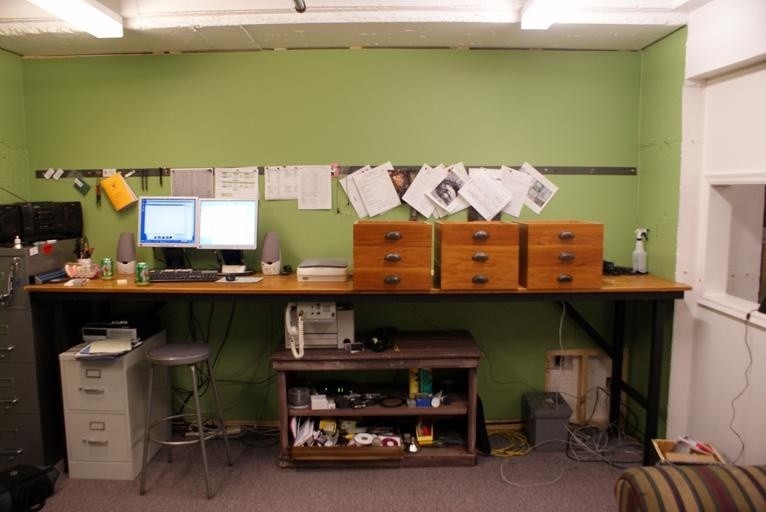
[63,262,98,278]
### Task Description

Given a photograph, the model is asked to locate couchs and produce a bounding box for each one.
[615,464,766,512]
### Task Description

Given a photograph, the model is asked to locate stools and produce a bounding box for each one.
[139,344,233,499]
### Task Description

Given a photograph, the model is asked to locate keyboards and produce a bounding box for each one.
[147,269,218,282]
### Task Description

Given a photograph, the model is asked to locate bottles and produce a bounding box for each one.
[135,262,150,286]
[13,236,23,250]
[100,257,113,280]
[631,228,650,273]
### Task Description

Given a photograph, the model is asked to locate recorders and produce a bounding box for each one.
[0,188,83,248]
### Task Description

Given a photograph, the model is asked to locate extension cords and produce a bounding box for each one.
[184,424,242,436]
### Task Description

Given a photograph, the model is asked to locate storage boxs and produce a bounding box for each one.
[435,221,520,291]
[0,240,78,475]
[352,222,434,288]
[520,224,607,287]
[58,328,168,481]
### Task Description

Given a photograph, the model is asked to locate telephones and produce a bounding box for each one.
[284,301,335,359]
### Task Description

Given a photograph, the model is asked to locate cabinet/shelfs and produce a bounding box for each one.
[268,330,484,469]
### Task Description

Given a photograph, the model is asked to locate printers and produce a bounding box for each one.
[283,306,354,350]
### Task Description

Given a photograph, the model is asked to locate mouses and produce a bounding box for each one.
[226,274,235,281]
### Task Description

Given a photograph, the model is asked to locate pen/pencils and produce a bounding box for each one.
[72,237,93,258]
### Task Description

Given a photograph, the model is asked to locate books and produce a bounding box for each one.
[100,174,139,213]
[291,417,314,449]
[669,435,715,456]
[75,339,143,360]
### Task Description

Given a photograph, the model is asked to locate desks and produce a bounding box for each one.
[23,271,694,472]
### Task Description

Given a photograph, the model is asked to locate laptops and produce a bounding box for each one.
[197,198,258,275]
[137,196,199,267]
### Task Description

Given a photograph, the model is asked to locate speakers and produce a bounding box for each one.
[115,232,136,275]
[259,231,281,276]
